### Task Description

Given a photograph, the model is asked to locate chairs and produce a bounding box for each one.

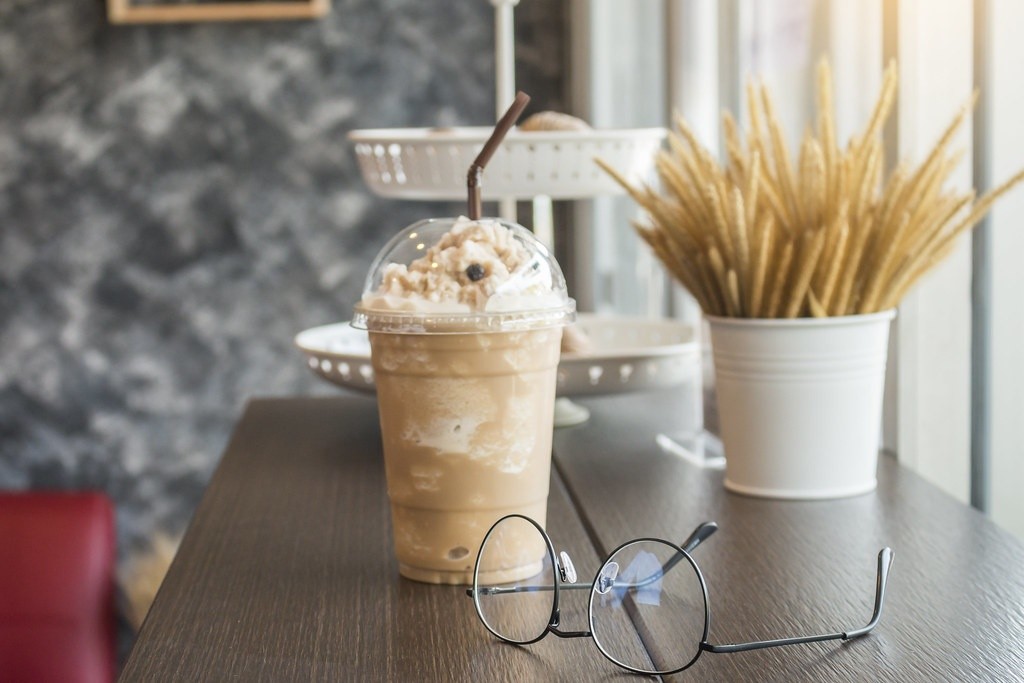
[0,487,117,683]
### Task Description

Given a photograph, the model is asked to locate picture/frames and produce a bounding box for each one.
[109,0,328,22]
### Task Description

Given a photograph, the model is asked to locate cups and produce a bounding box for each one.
[351,216,577,586]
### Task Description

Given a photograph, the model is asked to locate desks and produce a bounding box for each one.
[123,397,1024,683]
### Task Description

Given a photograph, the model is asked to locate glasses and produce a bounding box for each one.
[467,513,894,676]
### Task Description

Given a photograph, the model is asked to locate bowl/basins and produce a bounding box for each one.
[702,308,898,499]
